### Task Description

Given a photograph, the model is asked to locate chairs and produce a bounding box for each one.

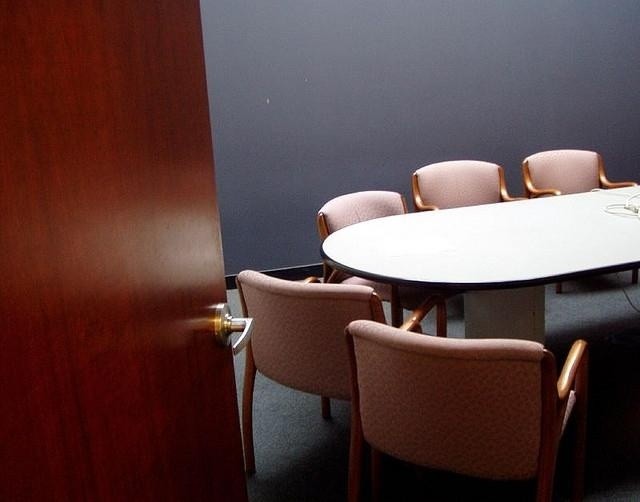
[314,190,428,315]
[409,158,531,211]
[519,149,640,294]
[236,273,452,502]
[346,292,592,502]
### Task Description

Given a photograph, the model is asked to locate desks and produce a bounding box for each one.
[319,189,640,356]
